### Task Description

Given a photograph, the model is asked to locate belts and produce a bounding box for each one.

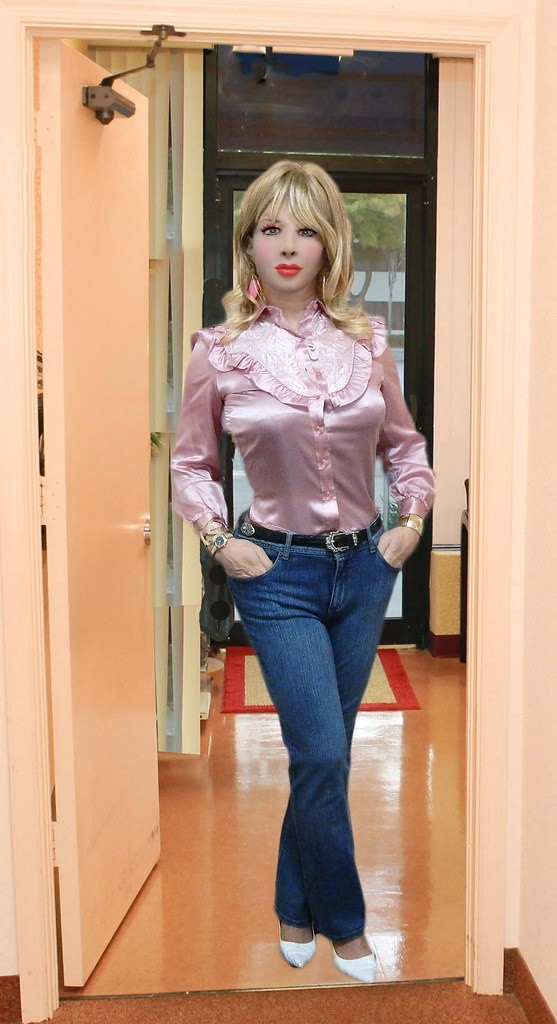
[238,515,383,552]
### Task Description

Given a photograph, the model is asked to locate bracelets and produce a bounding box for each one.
[198,516,230,548]
[396,513,426,537]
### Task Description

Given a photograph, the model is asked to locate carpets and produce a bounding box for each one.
[221,646,420,712]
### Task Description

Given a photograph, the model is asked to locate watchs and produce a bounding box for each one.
[210,532,234,557]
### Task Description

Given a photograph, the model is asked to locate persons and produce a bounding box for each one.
[170,158,436,986]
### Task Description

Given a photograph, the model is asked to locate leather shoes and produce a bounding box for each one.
[329,934,375,984]
[277,914,316,968]
